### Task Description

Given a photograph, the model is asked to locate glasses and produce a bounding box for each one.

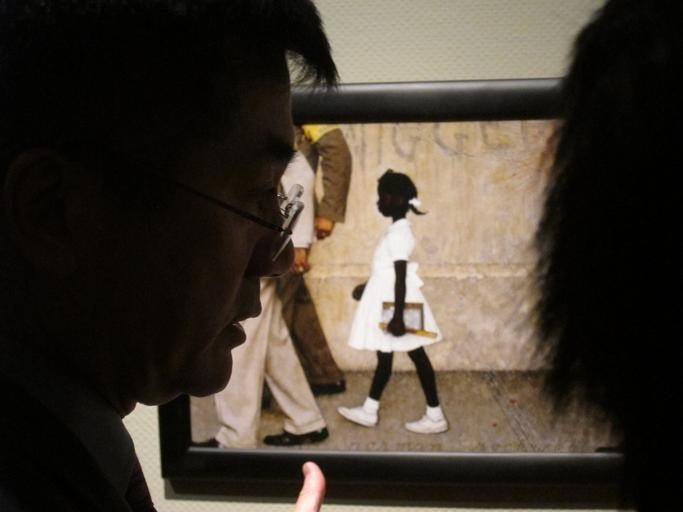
[131,156,306,264]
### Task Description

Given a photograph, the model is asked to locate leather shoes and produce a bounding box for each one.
[188,434,229,449]
[309,378,346,396]
[262,424,329,447]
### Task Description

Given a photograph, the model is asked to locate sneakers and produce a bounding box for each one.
[402,413,450,435]
[336,403,380,428]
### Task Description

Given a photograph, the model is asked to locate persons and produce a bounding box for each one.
[335,170,450,434]
[0,0,344,512]
[188,143,329,450]
[272,121,352,396]
[508,2,681,510]
